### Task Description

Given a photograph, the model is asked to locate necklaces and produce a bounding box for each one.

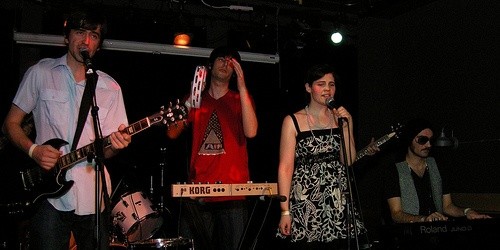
[305,105,334,148]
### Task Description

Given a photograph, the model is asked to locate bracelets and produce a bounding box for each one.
[282,210,292,217]
[27,143,38,158]
[463,207,471,215]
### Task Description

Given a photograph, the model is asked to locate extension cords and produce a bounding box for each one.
[229,5,253,11]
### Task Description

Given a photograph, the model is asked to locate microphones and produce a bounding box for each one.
[325,97,348,122]
[79,47,92,68]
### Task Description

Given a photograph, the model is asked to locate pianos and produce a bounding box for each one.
[380,217,500,250]
[171,180,280,197]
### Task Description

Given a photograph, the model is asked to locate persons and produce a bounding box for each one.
[275,64,380,250]
[386,126,493,224]
[168,43,258,250]
[2,13,133,250]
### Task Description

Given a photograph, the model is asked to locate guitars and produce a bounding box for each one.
[355,122,403,161]
[0,99,191,223]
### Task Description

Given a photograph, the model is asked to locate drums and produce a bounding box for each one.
[110,188,165,243]
[131,238,178,250]
[71,236,127,250]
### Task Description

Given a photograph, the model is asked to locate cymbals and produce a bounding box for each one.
[192,65,206,108]
